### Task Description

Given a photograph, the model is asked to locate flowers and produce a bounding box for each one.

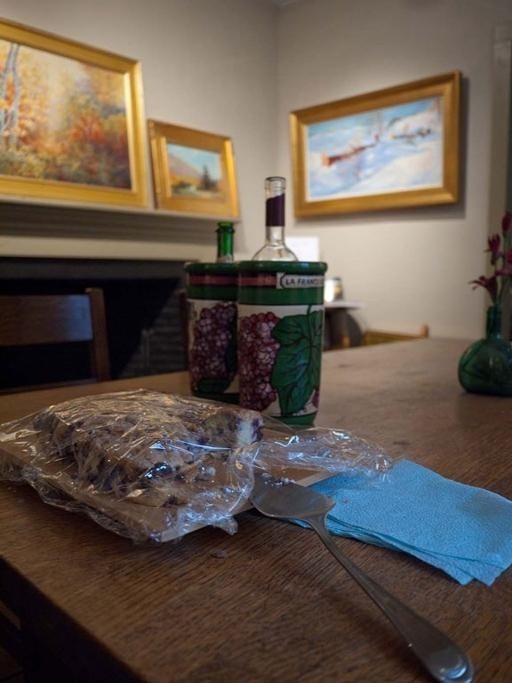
[467,209,512,305]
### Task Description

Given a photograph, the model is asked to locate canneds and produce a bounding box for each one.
[237,260,327,424]
[183,262,239,403]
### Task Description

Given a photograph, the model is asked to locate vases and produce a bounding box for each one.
[457,305,512,396]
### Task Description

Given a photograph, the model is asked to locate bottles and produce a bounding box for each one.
[215,175,298,260]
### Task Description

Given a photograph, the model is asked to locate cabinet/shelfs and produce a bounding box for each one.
[0,256,198,389]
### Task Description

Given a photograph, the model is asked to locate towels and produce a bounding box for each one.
[285,459,512,585]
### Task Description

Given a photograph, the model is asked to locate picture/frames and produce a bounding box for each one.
[147,119,239,216]
[289,71,459,217]
[1,16,150,207]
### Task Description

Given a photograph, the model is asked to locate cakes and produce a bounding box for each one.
[44,390,263,506]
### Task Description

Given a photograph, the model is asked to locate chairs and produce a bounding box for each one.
[0,287,110,395]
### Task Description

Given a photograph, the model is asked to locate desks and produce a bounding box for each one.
[0,337,512,683]
